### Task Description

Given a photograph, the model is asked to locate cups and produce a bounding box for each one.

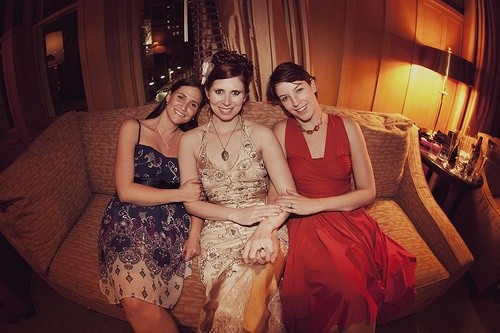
[428,141,442,160]
[440,144,450,162]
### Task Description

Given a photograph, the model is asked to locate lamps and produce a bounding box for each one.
[417,45,476,135]
[44,31,65,98]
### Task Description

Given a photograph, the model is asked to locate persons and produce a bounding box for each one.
[246,62,384,333]
[100,78,209,333]
[178,50,299,333]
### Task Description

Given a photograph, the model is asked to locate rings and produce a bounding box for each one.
[259,247,265,252]
[201,191,206,197]
[289,203,293,207]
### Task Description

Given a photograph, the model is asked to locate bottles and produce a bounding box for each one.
[467,136,483,172]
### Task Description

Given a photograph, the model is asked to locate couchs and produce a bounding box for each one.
[442,132,500,300]
[0,101,475,333]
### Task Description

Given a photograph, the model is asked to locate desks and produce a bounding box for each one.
[420,144,484,222]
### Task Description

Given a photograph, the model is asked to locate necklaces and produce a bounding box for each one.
[210,116,241,162]
[155,121,178,152]
[294,111,324,135]
